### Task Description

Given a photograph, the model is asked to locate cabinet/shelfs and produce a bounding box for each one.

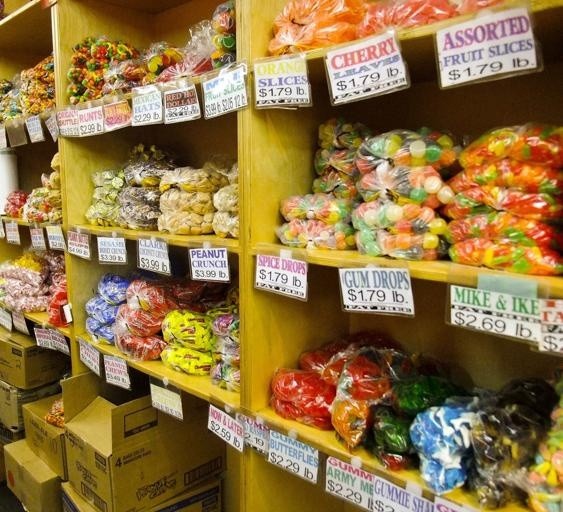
[244,0,563,511]
[54,0,244,419]
[0,0,72,341]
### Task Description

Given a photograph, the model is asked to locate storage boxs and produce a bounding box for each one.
[60,370,229,512]
[1,325,68,512]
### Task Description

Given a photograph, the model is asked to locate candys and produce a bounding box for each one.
[85,272,240,392]
[67,1,233,104]
[0,245,69,328]
[496,380,563,512]
[4,153,62,222]
[270,331,405,431]
[468,376,560,510]
[0,52,56,120]
[267,1,508,59]
[367,405,422,468]
[331,346,439,456]
[382,453,420,471]
[83,142,238,239]
[409,386,499,497]
[43,397,66,424]
[391,364,477,419]
